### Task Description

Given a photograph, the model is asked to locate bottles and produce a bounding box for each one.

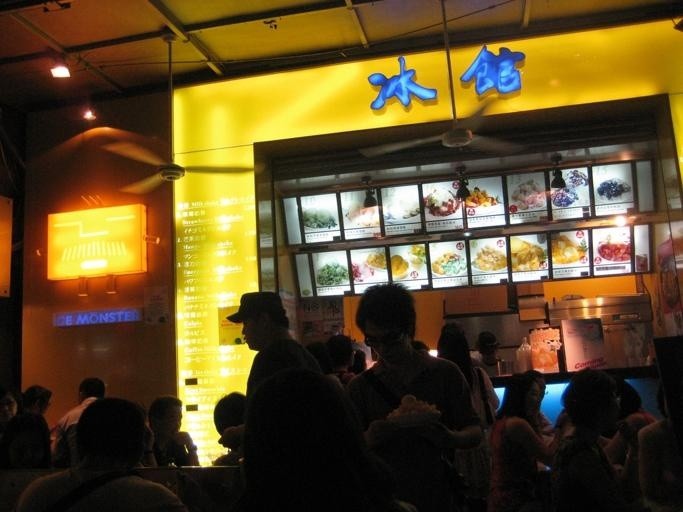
[516,337,531,372]
[624,327,640,355]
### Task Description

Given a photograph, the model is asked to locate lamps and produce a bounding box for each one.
[455,176,471,198]
[364,186,379,207]
[50,54,70,78]
[550,169,566,189]
[81,67,98,121]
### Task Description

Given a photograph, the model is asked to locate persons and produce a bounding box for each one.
[0,282,683,512]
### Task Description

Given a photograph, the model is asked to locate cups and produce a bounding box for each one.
[496,358,506,373]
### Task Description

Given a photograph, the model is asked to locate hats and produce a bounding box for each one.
[226,292,282,322]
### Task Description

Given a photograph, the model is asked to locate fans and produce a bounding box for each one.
[102,28,266,193]
[358,0,521,160]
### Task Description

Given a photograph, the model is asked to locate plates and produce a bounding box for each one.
[472,260,507,274]
[432,270,466,277]
[384,412,442,428]
[362,259,386,272]
